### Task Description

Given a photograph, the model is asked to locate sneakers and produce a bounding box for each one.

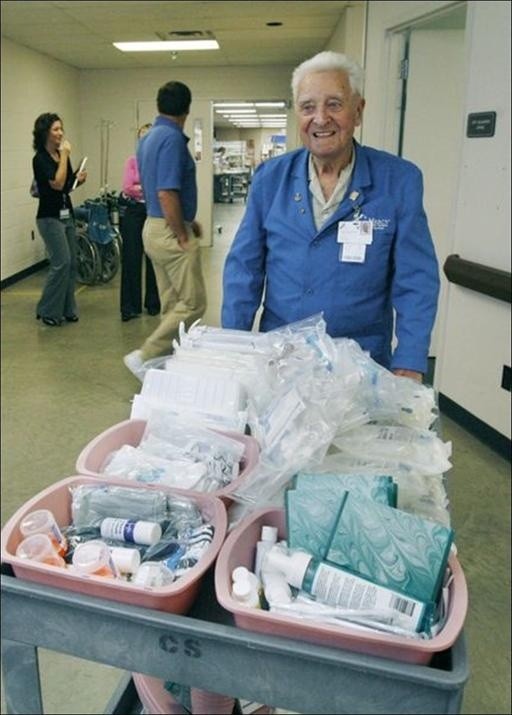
[123,351,146,383]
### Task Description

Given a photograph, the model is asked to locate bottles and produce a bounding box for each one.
[16,508,175,588]
[227,525,435,638]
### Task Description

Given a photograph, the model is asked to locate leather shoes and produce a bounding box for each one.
[64,315,79,322]
[36,314,61,326]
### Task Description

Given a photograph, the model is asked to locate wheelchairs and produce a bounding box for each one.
[67,184,124,285]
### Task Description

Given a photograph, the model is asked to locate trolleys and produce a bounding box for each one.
[220,165,252,205]
[0,381,474,715]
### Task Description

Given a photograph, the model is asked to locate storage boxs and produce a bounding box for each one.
[2,476,227,612]
[216,508,468,665]
[76,419,261,496]
[133,671,234,713]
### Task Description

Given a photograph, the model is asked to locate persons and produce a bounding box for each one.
[214,147,225,202]
[120,124,160,321]
[123,81,206,386]
[33,114,87,326]
[221,52,440,386]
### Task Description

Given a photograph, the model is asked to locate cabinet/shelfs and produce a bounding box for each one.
[214,167,251,203]
